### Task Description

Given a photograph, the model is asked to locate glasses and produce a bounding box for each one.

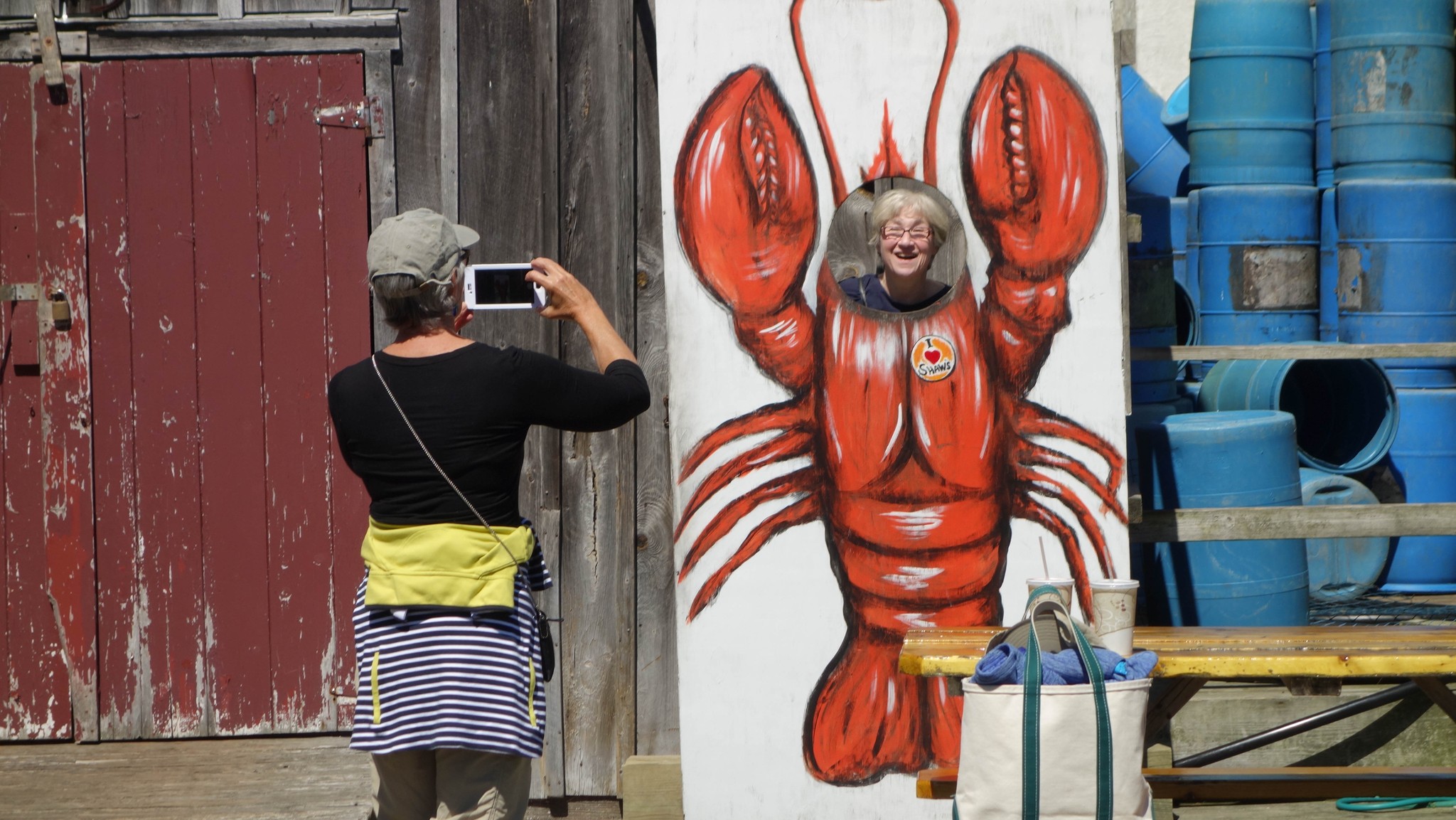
[881,226,932,240]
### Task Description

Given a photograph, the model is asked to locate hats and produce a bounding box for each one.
[366,208,480,299]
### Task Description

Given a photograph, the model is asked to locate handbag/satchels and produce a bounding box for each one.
[951,585,1154,820]
[537,609,555,681]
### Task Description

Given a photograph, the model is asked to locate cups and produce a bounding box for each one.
[1028,578,1075,614]
[1090,581,1138,657]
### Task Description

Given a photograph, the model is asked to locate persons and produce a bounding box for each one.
[837,189,952,313]
[326,208,652,820]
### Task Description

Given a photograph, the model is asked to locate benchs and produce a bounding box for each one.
[898,624,1456,802]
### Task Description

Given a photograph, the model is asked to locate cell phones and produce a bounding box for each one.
[463,263,546,310]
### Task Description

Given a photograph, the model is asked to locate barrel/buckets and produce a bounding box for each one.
[1111,0,1456,626]
